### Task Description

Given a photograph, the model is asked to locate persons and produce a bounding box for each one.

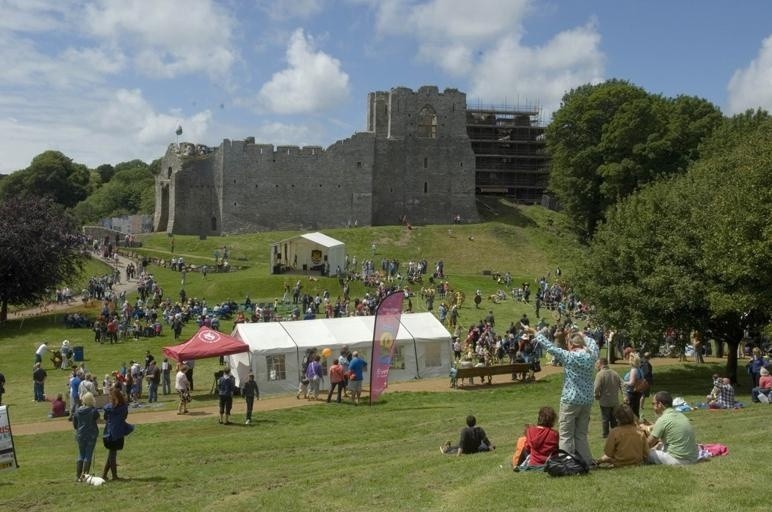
[0,372,6,406]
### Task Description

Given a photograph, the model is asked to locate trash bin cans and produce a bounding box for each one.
[362,261,375,274]
[73,347,83,361]
[382,261,399,273]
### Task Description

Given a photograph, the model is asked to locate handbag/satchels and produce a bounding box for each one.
[543,447,590,478]
[633,368,650,394]
[312,375,322,384]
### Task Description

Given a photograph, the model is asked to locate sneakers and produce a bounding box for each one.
[219,419,232,425]
[439,440,452,456]
[245,419,251,425]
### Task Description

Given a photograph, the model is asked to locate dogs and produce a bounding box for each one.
[78,474,106,486]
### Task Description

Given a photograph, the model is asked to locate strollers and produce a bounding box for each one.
[50,351,75,368]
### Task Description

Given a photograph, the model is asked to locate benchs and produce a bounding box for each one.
[452,363,530,390]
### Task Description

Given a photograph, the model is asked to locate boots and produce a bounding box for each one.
[110,464,120,481]
[75,459,91,483]
[102,463,110,482]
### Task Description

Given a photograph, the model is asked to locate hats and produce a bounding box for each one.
[63,340,70,345]
[520,333,529,340]
